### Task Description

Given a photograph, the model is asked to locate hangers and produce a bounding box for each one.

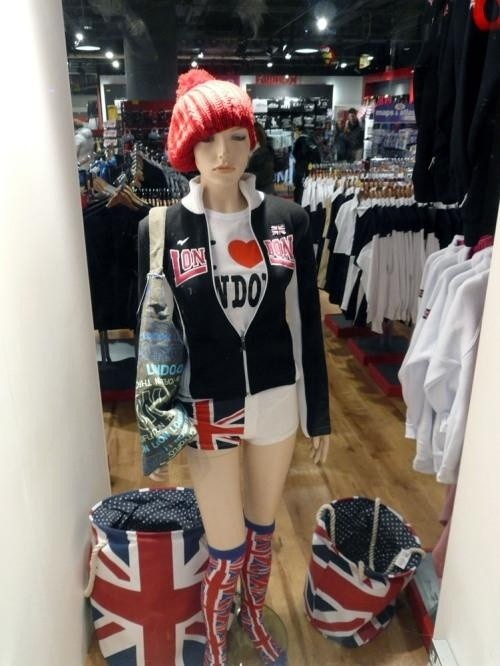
[79,143,181,210]
[310,158,413,198]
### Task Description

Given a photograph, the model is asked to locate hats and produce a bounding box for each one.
[166,68,260,174]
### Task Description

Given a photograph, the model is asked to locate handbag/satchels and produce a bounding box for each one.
[134,273,199,477]
[84,486,208,665]
[303,497,425,648]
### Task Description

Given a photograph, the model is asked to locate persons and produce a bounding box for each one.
[72,117,95,173]
[247,122,277,195]
[290,108,364,203]
[129,69,331,665]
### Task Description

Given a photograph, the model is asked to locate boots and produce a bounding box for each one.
[236,511,288,666]
[200,540,248,666]
